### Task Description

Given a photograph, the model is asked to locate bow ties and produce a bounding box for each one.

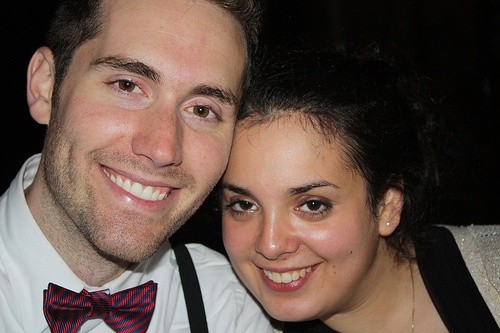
[43,279,158,332]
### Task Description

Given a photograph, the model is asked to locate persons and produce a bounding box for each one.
[222,45,500,333]
[1,0,287,333]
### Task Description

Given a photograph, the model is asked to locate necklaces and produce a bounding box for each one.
[405,249,416,333]
